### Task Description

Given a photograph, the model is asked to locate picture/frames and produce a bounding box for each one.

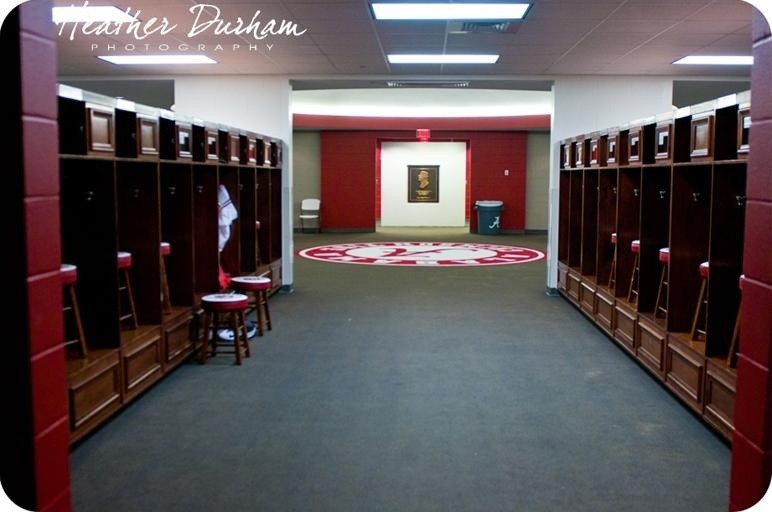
[407,165,439,203]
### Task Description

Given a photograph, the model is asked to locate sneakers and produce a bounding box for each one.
[218,323,256,341]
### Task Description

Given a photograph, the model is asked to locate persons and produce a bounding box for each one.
[416,170,432,190]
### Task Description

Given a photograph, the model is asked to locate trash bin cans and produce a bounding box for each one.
[474,200,503,235]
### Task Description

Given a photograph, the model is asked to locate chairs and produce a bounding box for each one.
[299,198,321,233]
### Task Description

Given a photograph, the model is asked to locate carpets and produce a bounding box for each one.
[296,240,547,267]
